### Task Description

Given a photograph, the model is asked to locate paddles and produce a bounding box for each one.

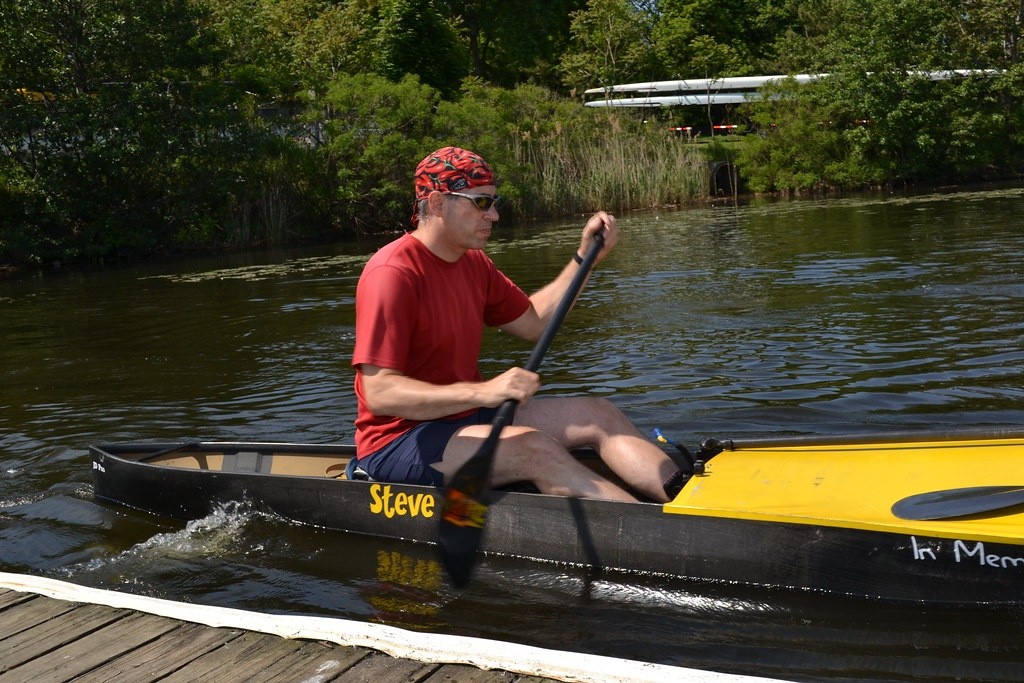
[433,219,607,584]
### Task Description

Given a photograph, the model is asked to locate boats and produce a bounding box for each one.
[88,425,1023,612]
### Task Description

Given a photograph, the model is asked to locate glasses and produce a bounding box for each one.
[444,189,500,212]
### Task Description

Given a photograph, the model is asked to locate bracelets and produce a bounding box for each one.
[573,253,584,265]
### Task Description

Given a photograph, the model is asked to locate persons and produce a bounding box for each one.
[355,145,681,504]
